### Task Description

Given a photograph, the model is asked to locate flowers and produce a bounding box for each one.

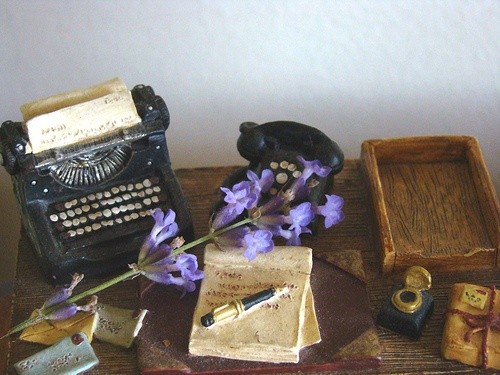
[0,155,345,340]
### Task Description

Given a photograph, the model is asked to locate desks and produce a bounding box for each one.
[6,163,500,375]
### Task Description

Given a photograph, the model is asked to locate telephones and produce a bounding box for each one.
[0,84,195,285]
[208,118,345,241]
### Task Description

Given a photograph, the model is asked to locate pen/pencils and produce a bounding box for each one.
[200,285,290,327]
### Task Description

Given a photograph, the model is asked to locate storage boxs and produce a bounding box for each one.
[362,136,500,276]
